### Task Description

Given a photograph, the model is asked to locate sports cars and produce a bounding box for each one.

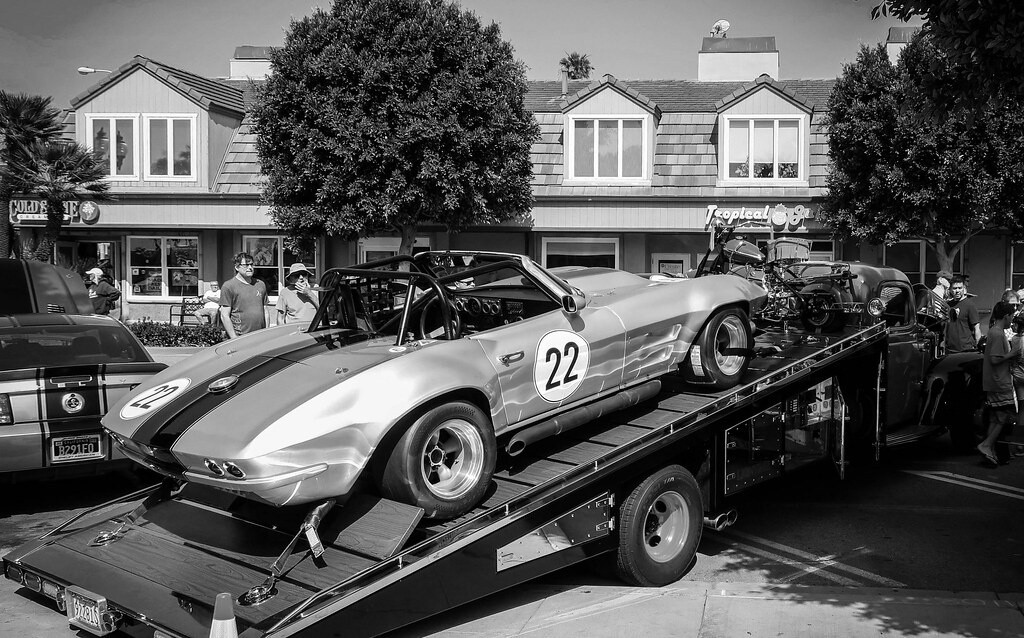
[98,248,770,520]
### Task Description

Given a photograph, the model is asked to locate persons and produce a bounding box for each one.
[275,263,319,326]
[219,251,270,341]
[974,300,1023,468]
[929,269,1024,433]
[86,268,121,315]
[192,281,222,327]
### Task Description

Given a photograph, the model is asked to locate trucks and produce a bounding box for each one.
[0,261,995,638]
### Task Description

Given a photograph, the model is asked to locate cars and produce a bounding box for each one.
[0,258,170,484]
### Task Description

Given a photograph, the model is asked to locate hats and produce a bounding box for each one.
[285,262,314,281]
[936,271,953,280]
[85,268,103,275]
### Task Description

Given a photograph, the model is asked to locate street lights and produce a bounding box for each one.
[78,65,112,75]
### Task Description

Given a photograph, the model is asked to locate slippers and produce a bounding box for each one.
[976,448,998,466]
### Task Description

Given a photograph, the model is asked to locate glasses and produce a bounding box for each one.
[1012,304,1019,307]
[965,280,969,282]
[290,273,307,278]
[240,263,253,267]
[459,280,475,285]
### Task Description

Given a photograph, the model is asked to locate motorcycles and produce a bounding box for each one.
[694,203,857,336]
